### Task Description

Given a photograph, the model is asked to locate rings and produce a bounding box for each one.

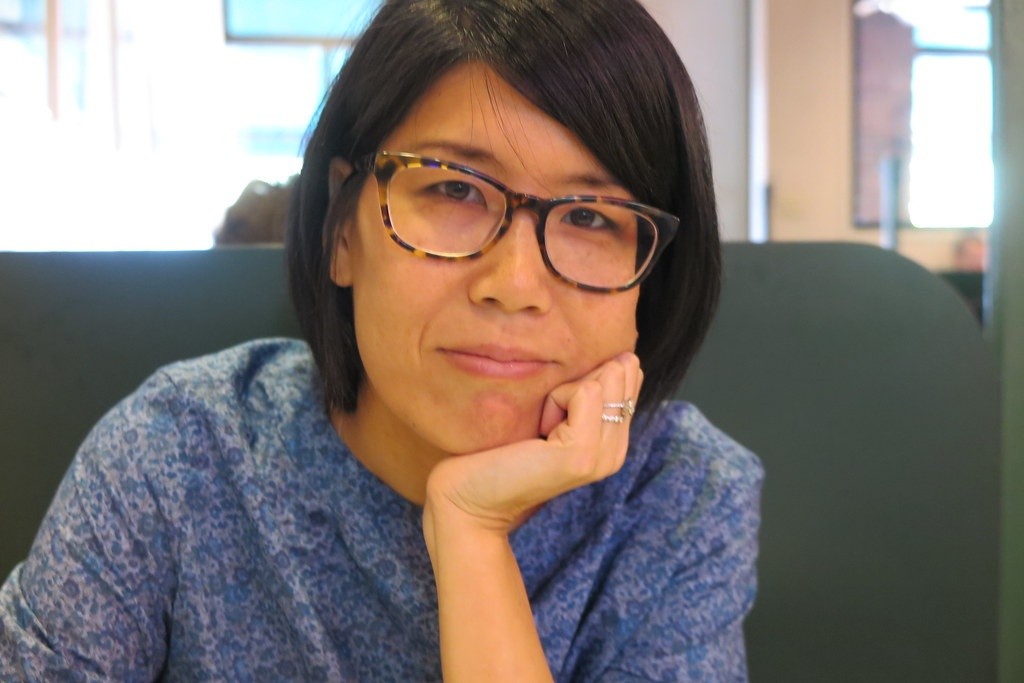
[605,400,635,416]
[602,415,625,422]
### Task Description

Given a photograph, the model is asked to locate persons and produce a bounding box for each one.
[0,0,764,683]
[215,173,301,244]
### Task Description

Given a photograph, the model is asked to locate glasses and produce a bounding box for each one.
[353,150,682,294]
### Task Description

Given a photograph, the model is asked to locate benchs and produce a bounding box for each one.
[1,241,1003,680]
